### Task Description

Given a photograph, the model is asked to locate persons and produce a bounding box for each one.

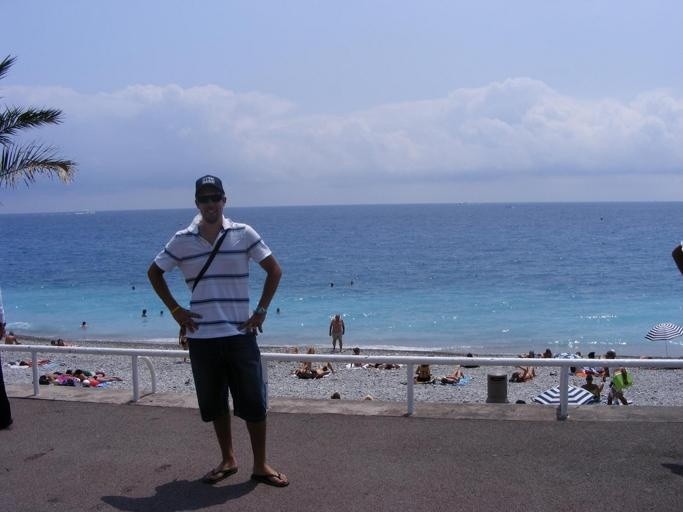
[352,348,364,367]
[375,363,400,370]
[0,322,122,390]
[460,353,480,369]
[544,349,552,358]
[295,348,334,379]
[510,366,535,382]
[327,313,346,353]
[416,365,430,382]
[147,173,290,490]
[140,309,147,317]
[441,369,465,384]
[581,350,628,404]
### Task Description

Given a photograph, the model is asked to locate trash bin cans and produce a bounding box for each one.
[487,371,508,404]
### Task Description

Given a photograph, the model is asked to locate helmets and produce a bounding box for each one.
[604,350,616,357]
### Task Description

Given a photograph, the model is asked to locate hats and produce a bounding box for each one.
[196,175,223,193]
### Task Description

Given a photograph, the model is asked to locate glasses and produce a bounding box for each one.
[196,192,222,203]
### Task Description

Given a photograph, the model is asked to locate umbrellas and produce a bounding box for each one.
[642,322,681,357]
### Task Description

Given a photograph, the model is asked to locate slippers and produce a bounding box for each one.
[202,467,238,484]
[251,471,289,487]
